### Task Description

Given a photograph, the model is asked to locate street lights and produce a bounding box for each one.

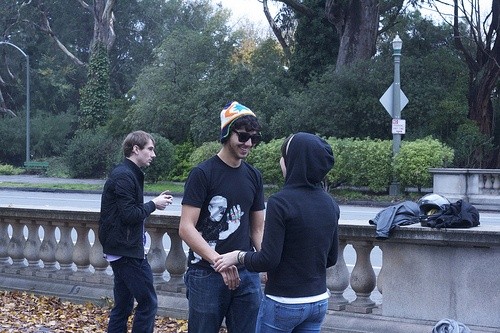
[389,31,403,196]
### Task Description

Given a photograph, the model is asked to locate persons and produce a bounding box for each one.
[214,132,340,333]
[178,100,265,333]
[98,129,172,333]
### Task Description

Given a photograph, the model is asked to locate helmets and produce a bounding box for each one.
[418,193,451,226]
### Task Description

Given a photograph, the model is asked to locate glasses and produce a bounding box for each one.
[232,129,261,146]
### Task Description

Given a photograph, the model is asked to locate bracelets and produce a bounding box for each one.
[238,251,246,265]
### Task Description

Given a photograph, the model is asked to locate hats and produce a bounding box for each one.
[220,100,256,144]
